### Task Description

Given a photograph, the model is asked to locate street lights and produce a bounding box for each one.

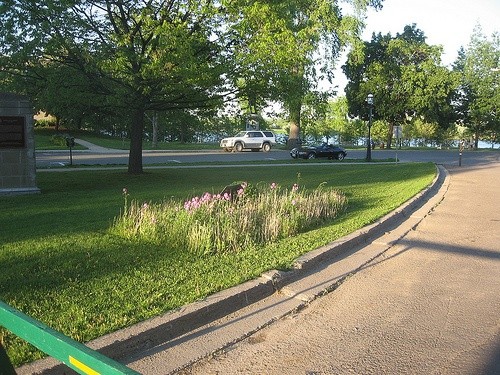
[366,94,373,161]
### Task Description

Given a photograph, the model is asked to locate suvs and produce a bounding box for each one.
[220,131,276,152]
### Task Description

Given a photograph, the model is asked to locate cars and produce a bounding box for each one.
[290,141,347,160]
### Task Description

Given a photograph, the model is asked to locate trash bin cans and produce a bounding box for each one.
[380,142,384,149]
